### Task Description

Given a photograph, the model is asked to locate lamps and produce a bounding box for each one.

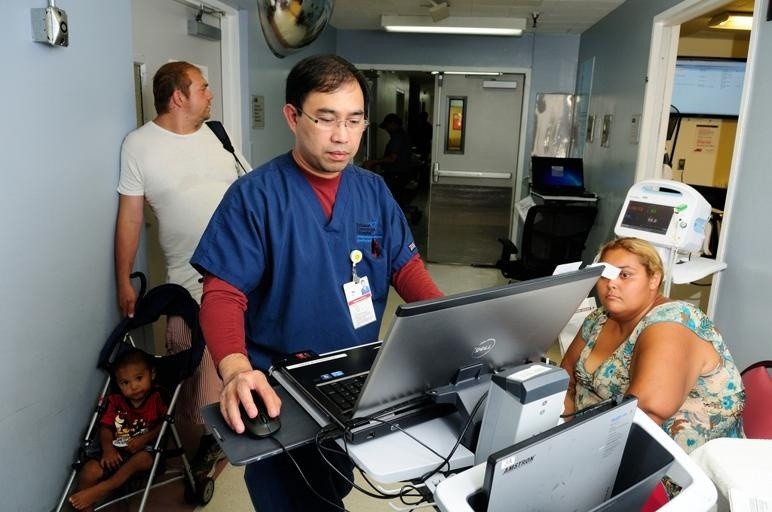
[380,12,527,39]
[709,13,753,31]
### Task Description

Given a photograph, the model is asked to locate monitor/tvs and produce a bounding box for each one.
[670,56,746,118]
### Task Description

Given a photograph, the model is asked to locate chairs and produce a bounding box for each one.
[740,361,772,440]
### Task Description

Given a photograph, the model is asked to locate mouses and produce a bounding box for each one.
[239,397,283,439]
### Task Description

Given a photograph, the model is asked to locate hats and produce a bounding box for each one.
[378,114,404,129]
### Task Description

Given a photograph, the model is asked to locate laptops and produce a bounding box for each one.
[278,260,607,448]
[530,155,600,202]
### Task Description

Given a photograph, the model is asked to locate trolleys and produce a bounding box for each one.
[48,272,215,510]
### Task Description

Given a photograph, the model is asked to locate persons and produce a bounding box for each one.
[113,60,255,480]
[187,52,446,512]
[68,350,175,512]
[359,279,369,295]
[415,111,432,171]
[558,235,748,512]
[360,110,423,225]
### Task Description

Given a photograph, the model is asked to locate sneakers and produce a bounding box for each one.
[180,432,228,484]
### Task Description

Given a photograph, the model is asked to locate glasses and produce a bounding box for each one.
[288,100,372,131]
